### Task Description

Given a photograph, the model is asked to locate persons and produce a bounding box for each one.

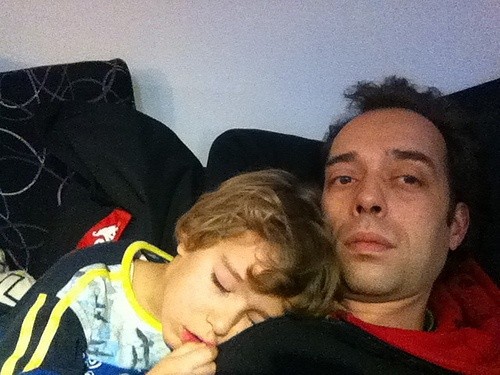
[209,75,500,375]
[1,169,343,374]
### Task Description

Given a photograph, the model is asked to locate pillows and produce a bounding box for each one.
[430,74,499,288]
[205,128,329,206]
[2,59,136,278]
